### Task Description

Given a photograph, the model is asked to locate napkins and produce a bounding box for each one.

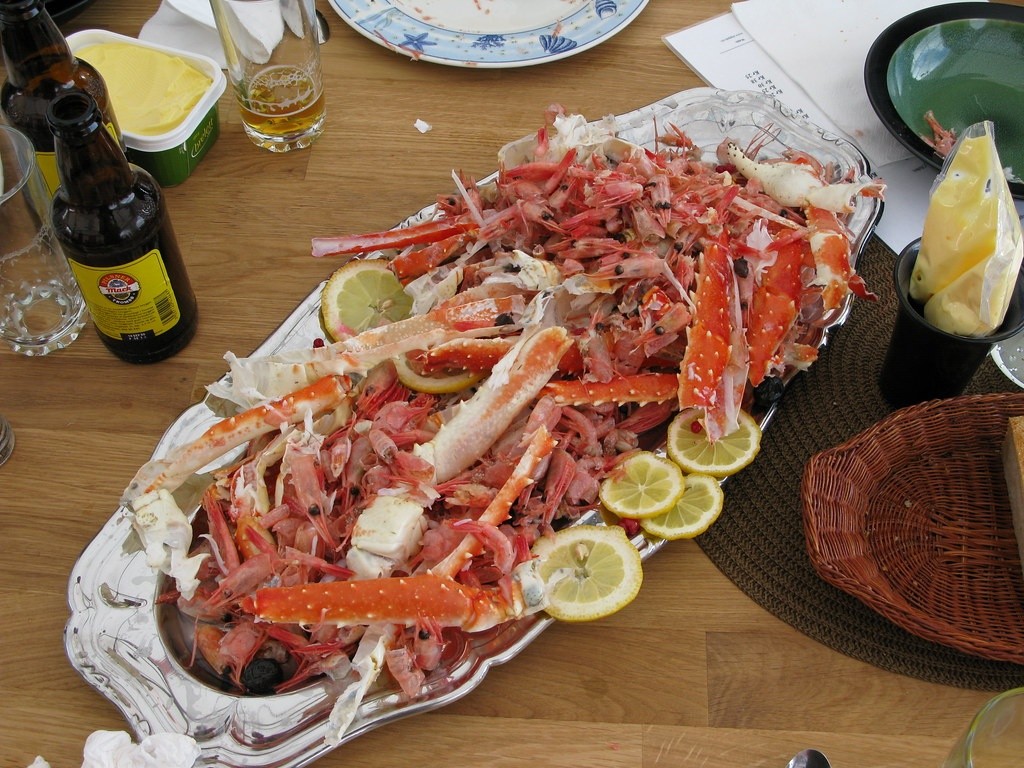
[729,0,988,168]
[137,0,286,70]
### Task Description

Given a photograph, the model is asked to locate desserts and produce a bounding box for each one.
[71,41,211,137]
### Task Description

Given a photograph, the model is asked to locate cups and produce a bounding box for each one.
[878,234,1023,409]
[0,127,88,356]
[211,0,327,152]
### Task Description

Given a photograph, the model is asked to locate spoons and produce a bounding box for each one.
[309,7,329,45]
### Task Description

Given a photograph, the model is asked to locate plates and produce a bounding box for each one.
[1,0,95,25]
[330,0,651,68]
[66,88,888,767]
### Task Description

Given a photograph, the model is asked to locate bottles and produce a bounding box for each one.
[45,92,198,365]
[1,2,127,252]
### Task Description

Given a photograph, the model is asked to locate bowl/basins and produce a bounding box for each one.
[867,4,1024,200]
[63,28,228,188]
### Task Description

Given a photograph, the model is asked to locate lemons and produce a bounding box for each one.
[321,258,415,343]
[638,471,723,540]
[665,403,762,477]
[524,524,642,623]
[598,450,684,520]
